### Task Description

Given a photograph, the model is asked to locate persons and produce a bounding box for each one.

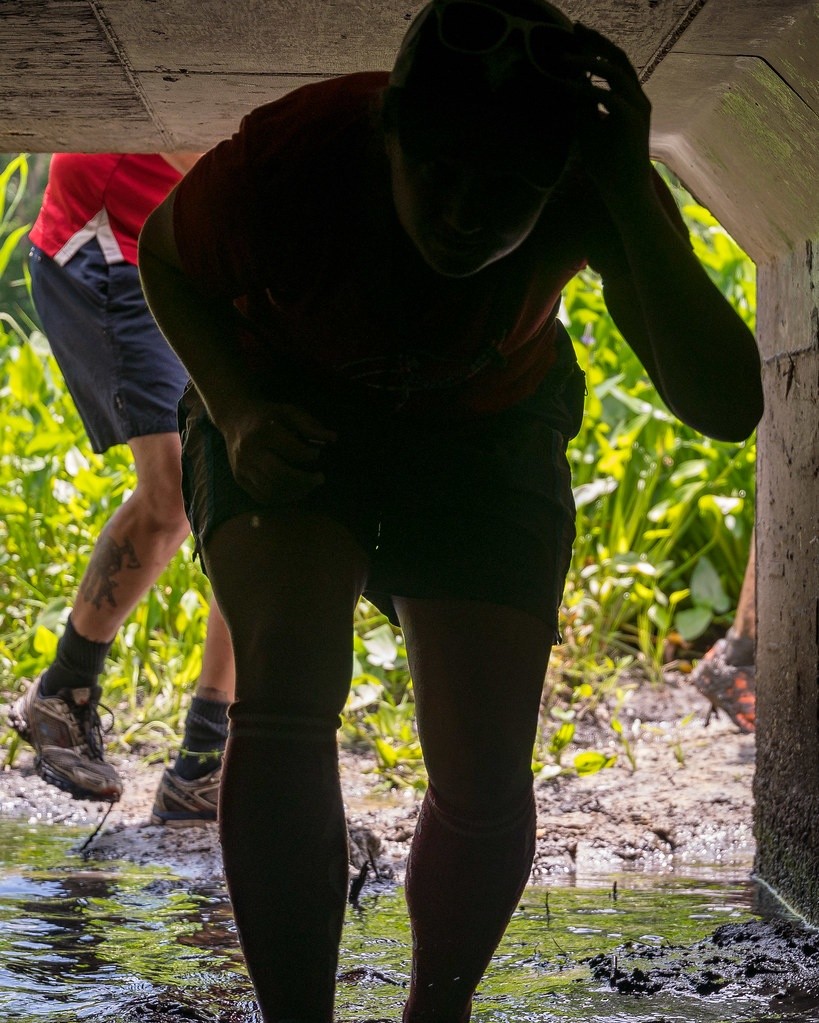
[689,526,756,735]
[136,0,765,1023]
[7,152,237,820]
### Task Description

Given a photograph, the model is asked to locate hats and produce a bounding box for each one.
[382,0,593,158]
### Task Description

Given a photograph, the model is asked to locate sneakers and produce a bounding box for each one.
[8,674,122,803]
[153,757,223,828]
